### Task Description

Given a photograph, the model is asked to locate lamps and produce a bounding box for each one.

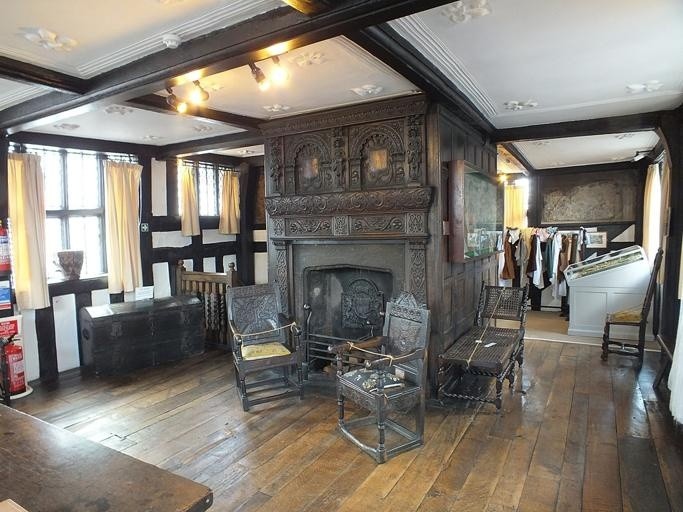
[633,150,654,162]
[165,87,188,116]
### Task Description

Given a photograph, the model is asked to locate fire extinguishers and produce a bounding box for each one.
[0,216,11,270]
[5,331,26,392]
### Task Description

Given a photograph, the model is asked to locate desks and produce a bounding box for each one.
[0,402,212,512]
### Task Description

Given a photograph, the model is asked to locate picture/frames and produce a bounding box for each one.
[585,230,608,249]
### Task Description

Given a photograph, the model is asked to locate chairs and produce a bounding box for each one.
[224,280,305,411]
[326,279,529,463]
[601,248,664,364]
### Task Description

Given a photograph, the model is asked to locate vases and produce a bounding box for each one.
[55,250,84,281]
